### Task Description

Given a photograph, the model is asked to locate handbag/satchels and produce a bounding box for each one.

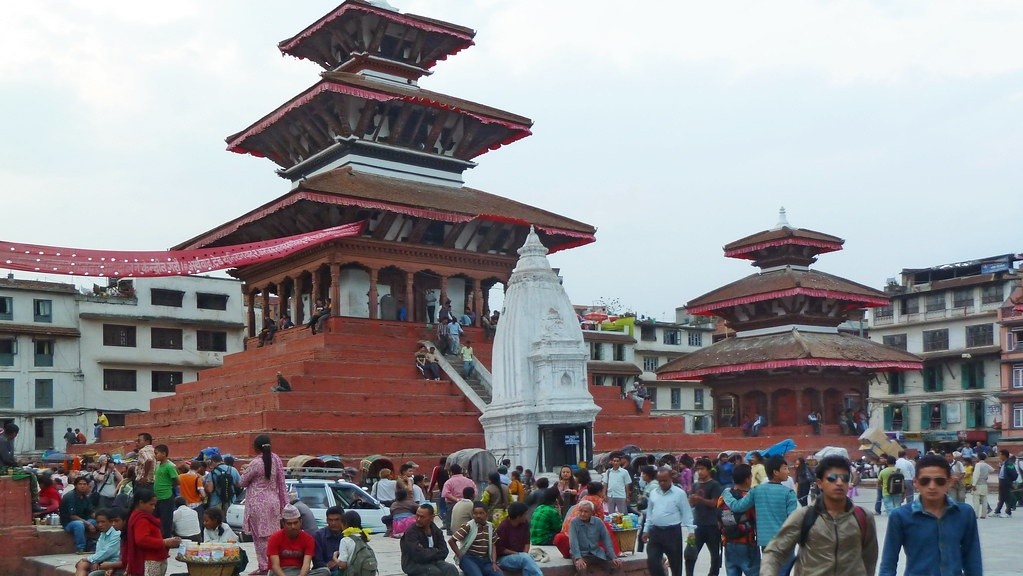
[90,491,100,505]
[511,492,519,503]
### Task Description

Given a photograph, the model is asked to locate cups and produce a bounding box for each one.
[8,470,13,475]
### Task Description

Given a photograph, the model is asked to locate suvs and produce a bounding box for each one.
[225,466,391,535]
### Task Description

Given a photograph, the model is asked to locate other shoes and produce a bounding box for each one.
[248,568,268,576]
[873,510,880,515]
[988,512,1002,517]
[999,513,1012,518]
[32,502,47,513]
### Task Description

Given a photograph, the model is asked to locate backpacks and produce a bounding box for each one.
[212,465,234,500]
[718,489,754,540]
[886,468,905,495]
[345,535,378,576]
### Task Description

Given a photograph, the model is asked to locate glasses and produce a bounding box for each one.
[919,476,948,486]
[823,473,849,483]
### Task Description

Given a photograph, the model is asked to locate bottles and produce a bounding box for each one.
[178,539,239,561]
[602,511,638,531]
[34,513,59,526]
[687,536,697,552]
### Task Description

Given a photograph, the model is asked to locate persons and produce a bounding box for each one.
[64,428,86,444]
[271,371,291,392]
[839,408,869,436]
[92,410,109,440]
[627,379,655,413]
[425,288,476,355]
[414,345,440,380]
[460,341,474,380]
[33,432,1023,576]
[256,312,295,348]
[729,411,764,437]
[808,410,822,435]
[0,423,47,512]
[306,297,331,335]
[481,309,500,340]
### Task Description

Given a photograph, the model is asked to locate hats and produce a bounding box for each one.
[282,504,301,520]
[406,461,419,468]
